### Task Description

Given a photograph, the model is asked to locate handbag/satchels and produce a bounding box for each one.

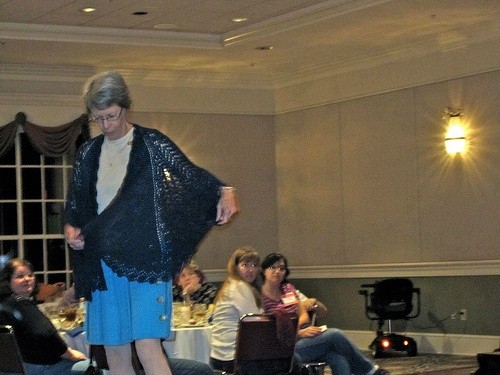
[83,344,104,375]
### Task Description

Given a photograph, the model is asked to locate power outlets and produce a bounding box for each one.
[461,309,468,321]
[450,313,456,319]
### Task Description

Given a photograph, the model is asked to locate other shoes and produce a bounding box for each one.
[373,369,390,375]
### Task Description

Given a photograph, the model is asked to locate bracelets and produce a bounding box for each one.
[217,186,237,196]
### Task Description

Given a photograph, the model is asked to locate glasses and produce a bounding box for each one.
[87,107,124,122]
[268,265,287,270]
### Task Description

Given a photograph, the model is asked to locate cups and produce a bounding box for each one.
[43,296,79,330]
[169,302,216,330]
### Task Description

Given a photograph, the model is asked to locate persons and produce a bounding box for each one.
[260,253,388,375]
[61,71,236,375]
[58,283,76,306]
[208,247,302,374]
[172,261,217,304]
[0,258,109,375]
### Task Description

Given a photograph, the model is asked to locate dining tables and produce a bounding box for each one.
[58,326,212,364]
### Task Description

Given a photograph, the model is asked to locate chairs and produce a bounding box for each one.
[358,278,421,356]
[0,325,27,375]
[212,312,299,375]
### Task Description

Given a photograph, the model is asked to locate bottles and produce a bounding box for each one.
[76,297,89,325]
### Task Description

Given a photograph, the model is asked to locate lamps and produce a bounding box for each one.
[442,105,469,156]
